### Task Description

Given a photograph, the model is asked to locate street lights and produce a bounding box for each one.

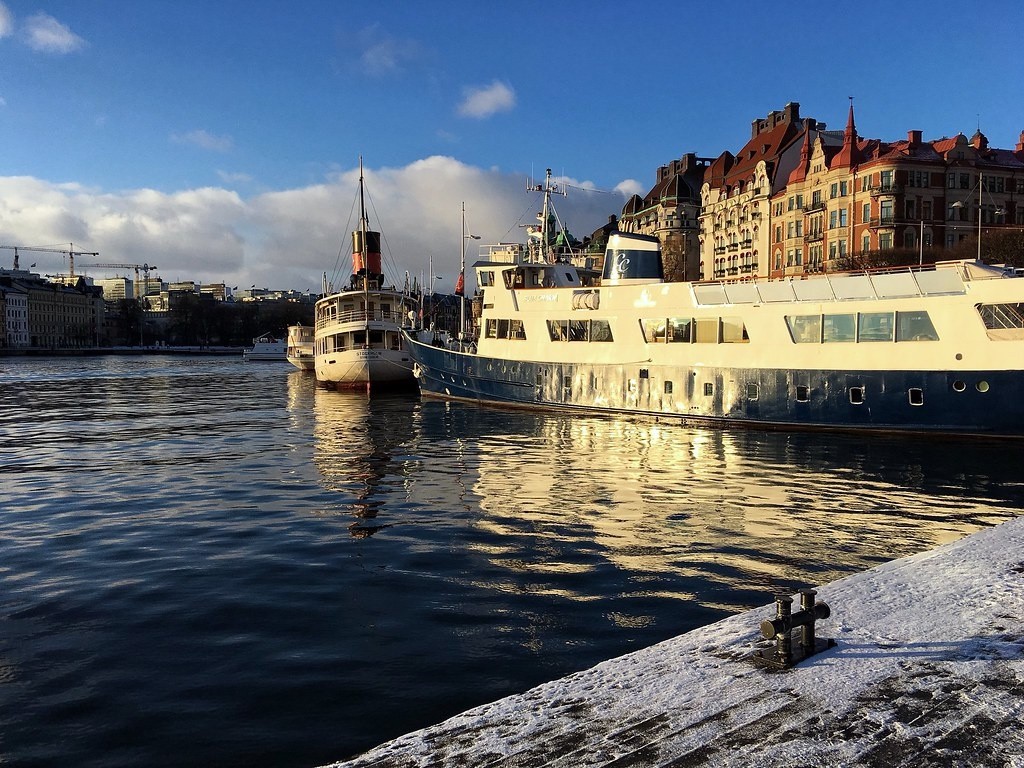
[460,199,482,330]
[428,254,443,293]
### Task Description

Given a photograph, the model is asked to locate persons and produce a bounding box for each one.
[431,338,445,348]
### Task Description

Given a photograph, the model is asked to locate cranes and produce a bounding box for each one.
[78,261,157,297]
[0,240,100,278]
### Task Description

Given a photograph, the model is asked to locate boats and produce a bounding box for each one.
[285,322,315,370]
[395,160,1024,447]
[241,333,287,361]
[314,149,421,384]
